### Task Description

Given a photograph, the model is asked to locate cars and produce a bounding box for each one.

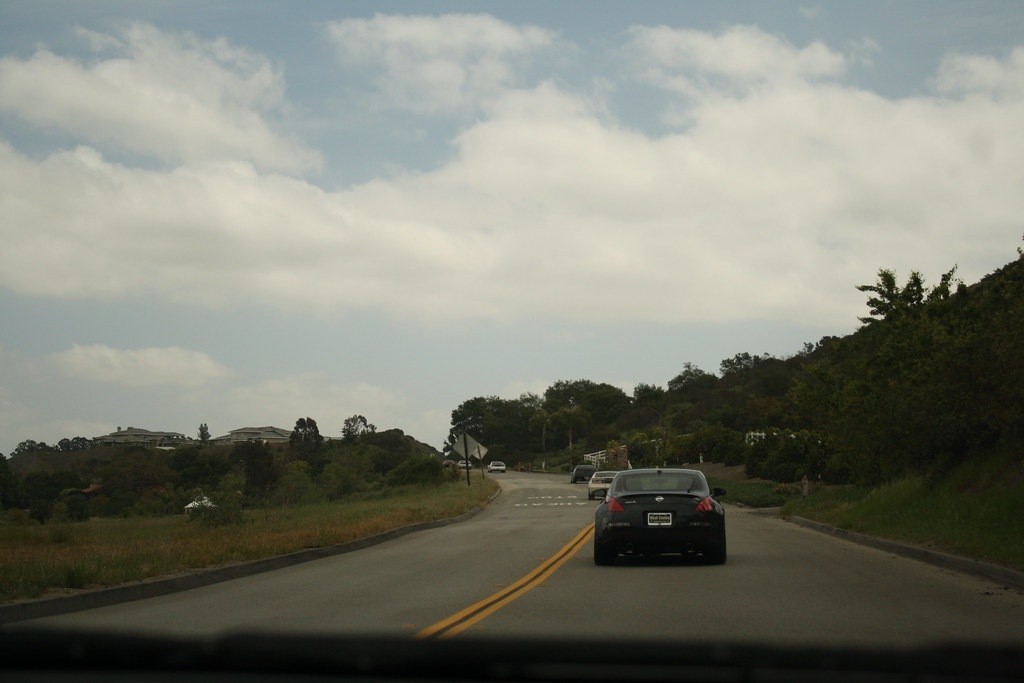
[444,460,472,470]
[489,461,505,473]
[572,465,618,500]
[594,469,726,566]
